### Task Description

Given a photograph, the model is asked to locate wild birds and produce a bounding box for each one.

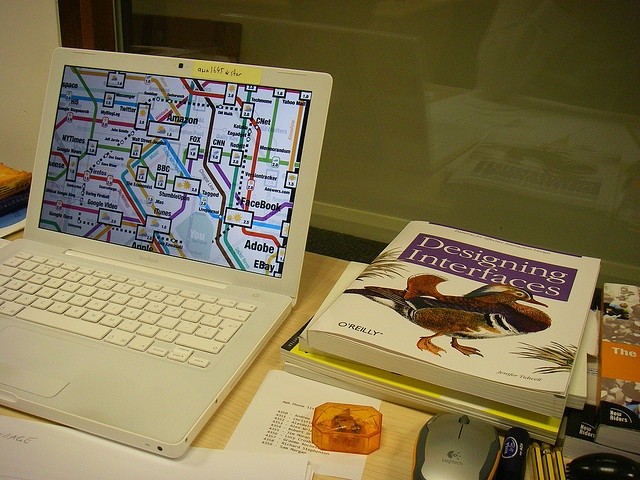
[343,276,553,355]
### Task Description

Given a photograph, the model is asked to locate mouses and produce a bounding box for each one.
[412,411,501,476]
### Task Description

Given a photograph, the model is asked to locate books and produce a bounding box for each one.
[0,163,32,217]
[446,145,623,216]
[280,219,640,464]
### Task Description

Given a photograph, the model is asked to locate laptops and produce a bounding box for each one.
[1,46,334,460]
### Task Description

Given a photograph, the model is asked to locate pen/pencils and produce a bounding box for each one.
[552,446,568,480]
[531,441,543,480]
[540,442,555,480]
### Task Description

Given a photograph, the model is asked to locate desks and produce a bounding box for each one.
[0,228,434,479]
